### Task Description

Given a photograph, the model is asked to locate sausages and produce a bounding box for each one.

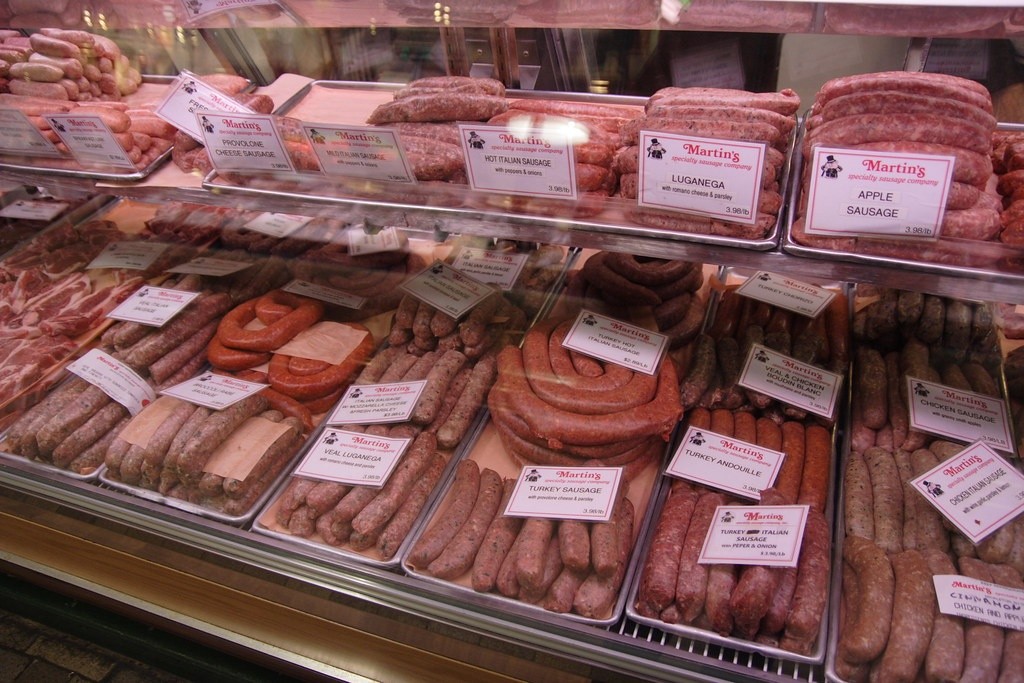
[0,26,1024,274]
[0,198,1024,683]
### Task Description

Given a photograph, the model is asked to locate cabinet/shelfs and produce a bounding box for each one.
[0,0,1024,682]
